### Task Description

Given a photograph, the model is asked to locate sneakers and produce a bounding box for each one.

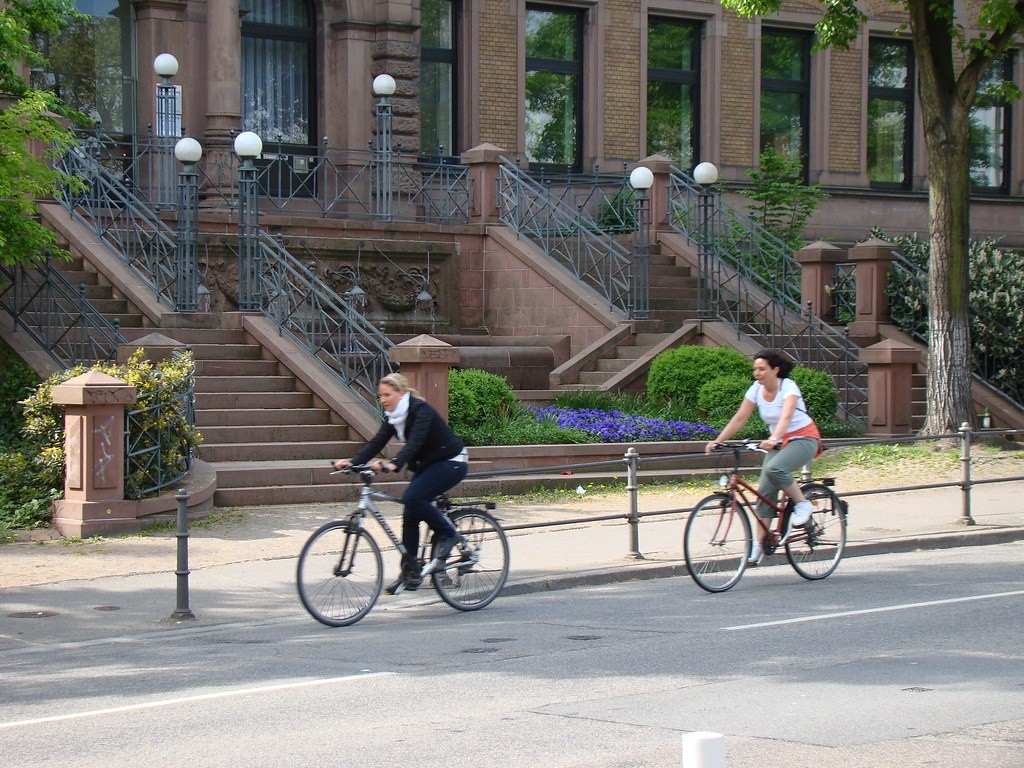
[793,500,813,526]
[741,545,765,565]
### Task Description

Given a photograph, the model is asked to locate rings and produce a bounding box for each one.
[766,447,770,448]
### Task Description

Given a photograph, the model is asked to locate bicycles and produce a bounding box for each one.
[298,461,509,627]
[683,438,849,592]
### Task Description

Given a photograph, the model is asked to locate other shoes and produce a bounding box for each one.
[388,572,408,595]
[437,533,460,558]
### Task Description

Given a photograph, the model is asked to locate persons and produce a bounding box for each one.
[334,373,469,594]
[705,348,822,563]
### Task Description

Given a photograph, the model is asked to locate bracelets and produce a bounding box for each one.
[769,436,778,441]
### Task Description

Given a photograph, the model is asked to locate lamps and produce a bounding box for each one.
[693,161,718,195]
[234,131,263,170]
[630,166,654,199]
[373,73,396,105]
[154,52,179,88]
[175,138,202,173]
